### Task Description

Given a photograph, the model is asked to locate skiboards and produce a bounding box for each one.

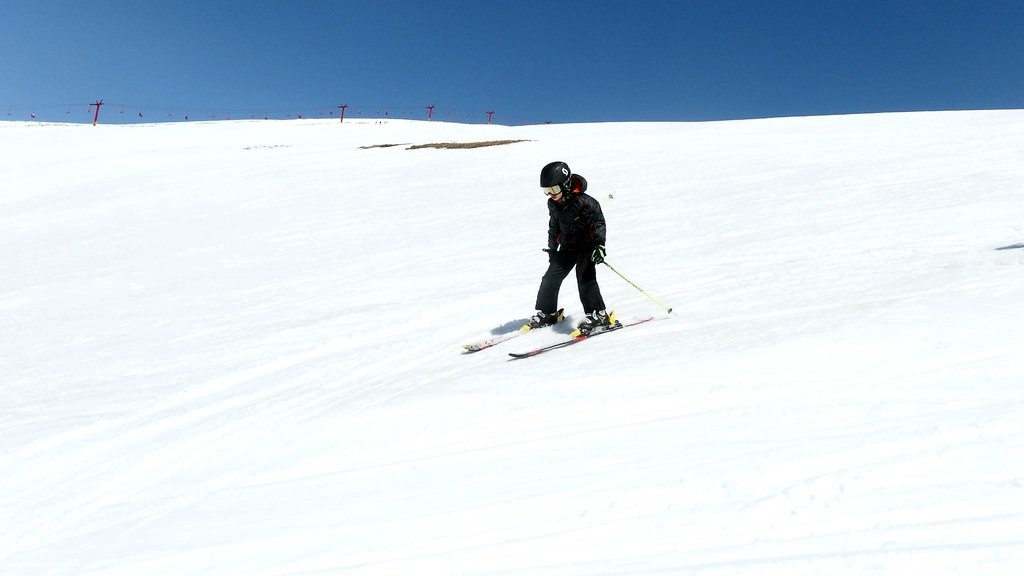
[462,308,654,358]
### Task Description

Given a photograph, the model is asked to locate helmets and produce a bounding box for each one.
[540,161,570,188]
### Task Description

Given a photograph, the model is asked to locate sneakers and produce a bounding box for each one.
[570,309,622,337]
[519,308,565,332]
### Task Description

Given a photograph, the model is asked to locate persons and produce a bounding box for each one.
[527,161,607,334]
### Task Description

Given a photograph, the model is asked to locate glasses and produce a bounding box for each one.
[541,184,562,196]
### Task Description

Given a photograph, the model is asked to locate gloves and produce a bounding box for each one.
[548,249,556,263]
[591,244,606,265]
